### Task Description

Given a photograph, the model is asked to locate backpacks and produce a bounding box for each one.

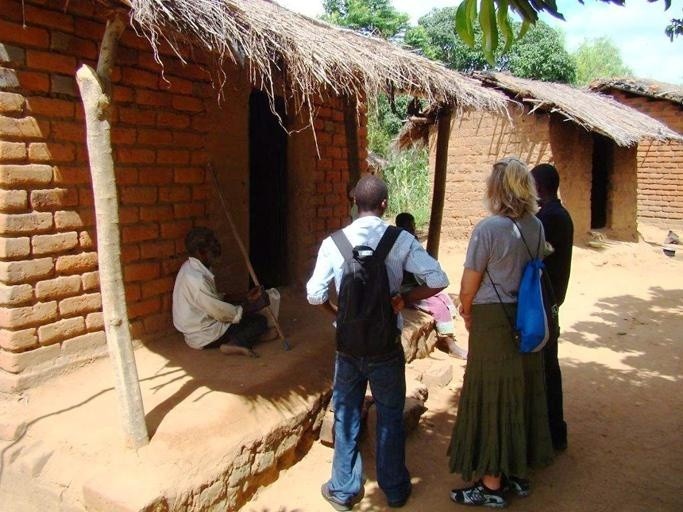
[329,225,404,358]
[484,215,550,355]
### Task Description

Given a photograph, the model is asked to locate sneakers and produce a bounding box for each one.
[501,473,530,497]
[320,482,353,512]
[449,478,508,510]
[386,482,412,507]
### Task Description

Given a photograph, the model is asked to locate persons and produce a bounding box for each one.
[306,174,450,512]
[445,154,558,509]
[529,160,575,452]
[172,226,271,359]
[394,211,468,359]
